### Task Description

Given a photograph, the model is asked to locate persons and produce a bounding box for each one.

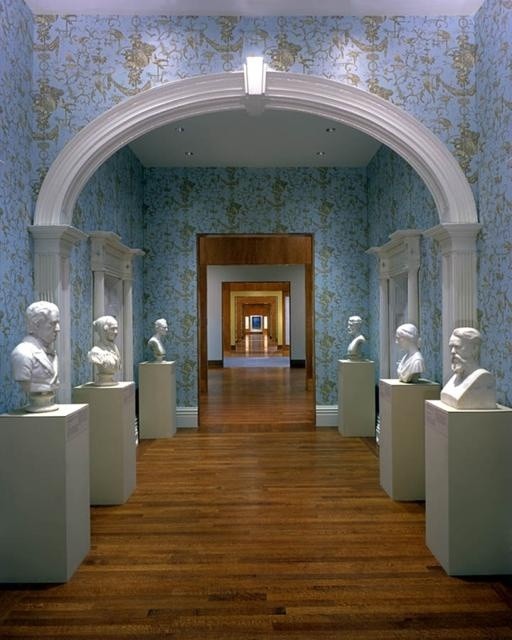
[441,326,497,409]
[395,323,425,383]
[87,315,122,386]
[10,300,61,413]
[148,318,168,363]
[347,316,366,361]
